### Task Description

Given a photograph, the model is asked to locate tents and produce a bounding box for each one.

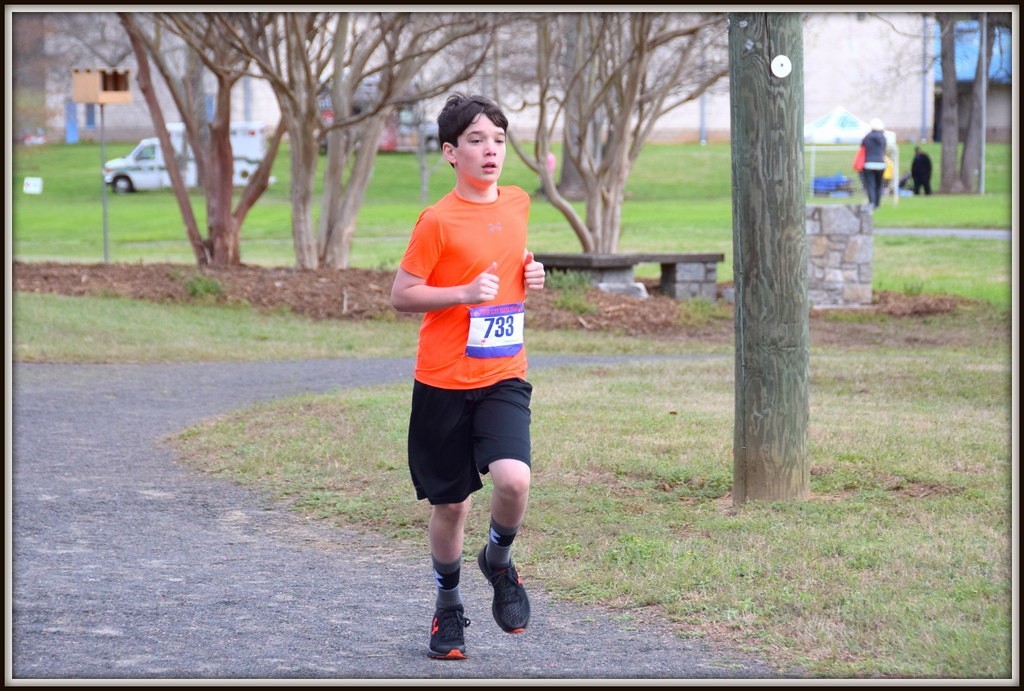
[804,106,899,203]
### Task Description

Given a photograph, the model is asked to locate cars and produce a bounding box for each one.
[425,121,444,155]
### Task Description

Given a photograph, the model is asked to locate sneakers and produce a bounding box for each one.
[427,603,471,660]
[478,543,530,634]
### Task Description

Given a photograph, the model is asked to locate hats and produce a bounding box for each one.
[870,118,884,131]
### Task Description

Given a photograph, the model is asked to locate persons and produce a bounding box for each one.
[912,146,933,195]
[861,119,886,206]
[390,93,545,658]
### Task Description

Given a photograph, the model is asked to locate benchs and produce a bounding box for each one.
[533,253,726,301]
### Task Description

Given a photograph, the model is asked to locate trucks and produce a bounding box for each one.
[100,124,272,193]
